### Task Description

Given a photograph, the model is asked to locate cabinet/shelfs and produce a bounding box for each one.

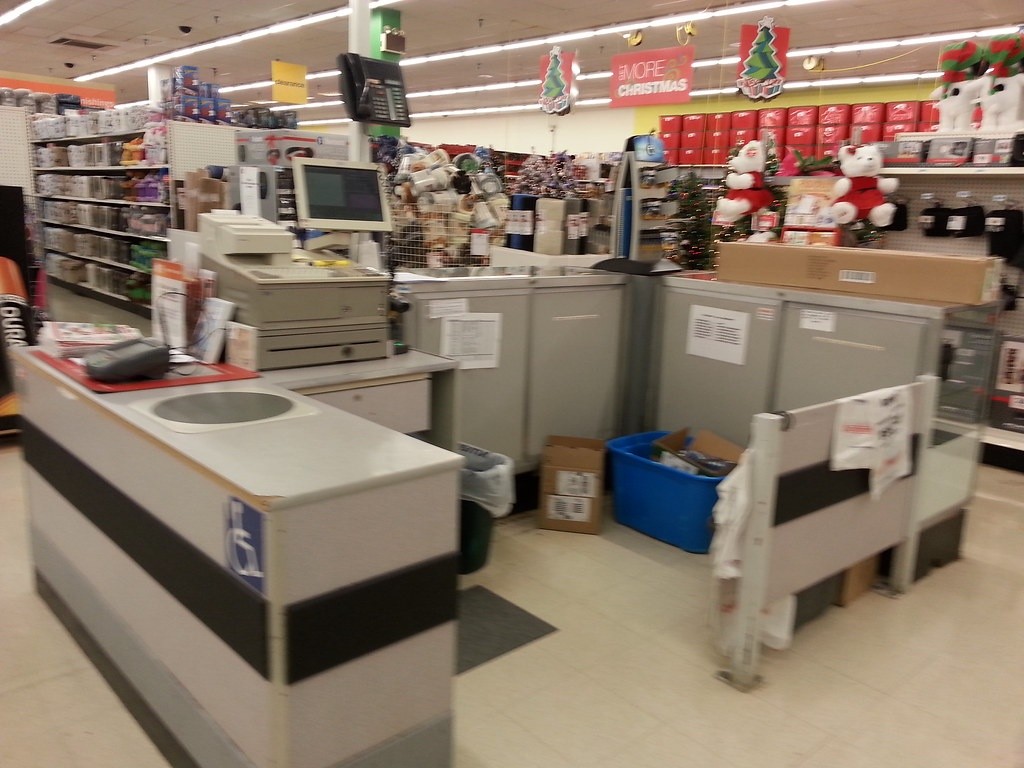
[661,293,928,456]
[583,146,625,253]
[31,124,174,319]
[627,154,727,256]
[412,285,627,470]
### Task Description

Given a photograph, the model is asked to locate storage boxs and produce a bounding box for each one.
[538,433,605,536]
[712,236,1002,306]
[605,430,727,555]
[656,99,939,165]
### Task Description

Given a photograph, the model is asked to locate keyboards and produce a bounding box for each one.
[244,266,393,284]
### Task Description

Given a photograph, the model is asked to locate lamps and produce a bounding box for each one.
[0,0,1024,116]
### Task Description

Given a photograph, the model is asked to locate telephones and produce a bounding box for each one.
[334,52,412,128]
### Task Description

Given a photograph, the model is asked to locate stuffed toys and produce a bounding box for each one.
[829,143,900,226]
[715,139,774,217]
[932,34,1024,133]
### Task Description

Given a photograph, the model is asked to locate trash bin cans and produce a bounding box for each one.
[458,440,516,576]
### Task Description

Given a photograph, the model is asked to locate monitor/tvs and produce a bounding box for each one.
[291,157,393,232]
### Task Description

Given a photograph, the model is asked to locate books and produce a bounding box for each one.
[37,257,257,374]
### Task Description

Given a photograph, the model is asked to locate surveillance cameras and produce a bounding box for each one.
[64,63,73,69]
[178,26,192,33]
